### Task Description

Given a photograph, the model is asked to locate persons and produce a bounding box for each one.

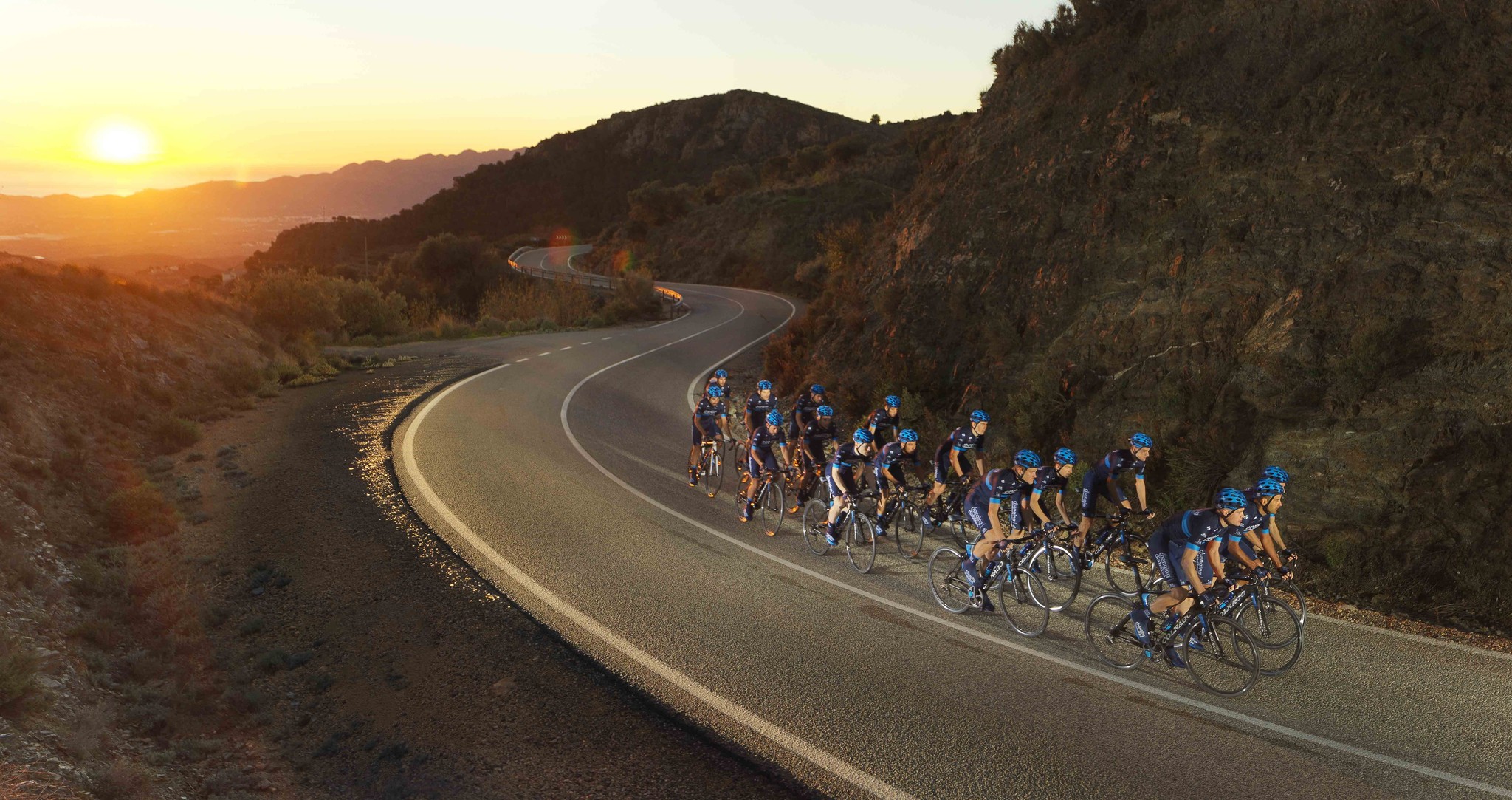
[1128,487,1247,668]
[920,410,990,530]
[853,395,901,485]
[785,384,829,470]
[1159,476,1293,651]
[1069,432,1155,577]
[703,369,732,447]
[744,379,778,469]
[794,405,840,508]
[742,411,794,520]
[961,449,1041,612]
[687,386,737,487]
[1217,464,1298,619]
[871,429,933,537]
[824,428,882,546]
[978,446,1080,579]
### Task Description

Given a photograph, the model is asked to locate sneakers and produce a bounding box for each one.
[1130,605,1150,645]
[687,463,963,545]
[1069,553,1084,580]
[1156,637,1187,669]
[972,583,996,611]
[1021,543,1041,574]
[962,555,984,590]
[1178,622,1206,653]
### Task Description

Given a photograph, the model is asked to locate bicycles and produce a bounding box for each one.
[686,392,1305,717]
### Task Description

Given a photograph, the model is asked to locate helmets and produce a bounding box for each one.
[1251,477,1285,498]
[756,379,773,392]
[969,409,992,424]
[851,428,875,447]
[882,395,902,409]
[706,386,721,399]
[1263,465,1289,483]
[808,383,825,395]
[714,368,728,380]
[1211,488,1247,511]
[1129,433,1154,449]
[1012,451,1041,471]
[764,412,784,428]
[1053,448,1077,468]
[896,429,919,444]
[814,405,834,418]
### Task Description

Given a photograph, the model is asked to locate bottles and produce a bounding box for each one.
[756,481,766,493]
[1096,531,1110,546]
[1020,541,1033,556]
[981,560,996,577]
[945,491,956,505]
[1161,612,1179,632]
[887,498,898,513]
[702,453,708,460]
[834,510,847,527]
